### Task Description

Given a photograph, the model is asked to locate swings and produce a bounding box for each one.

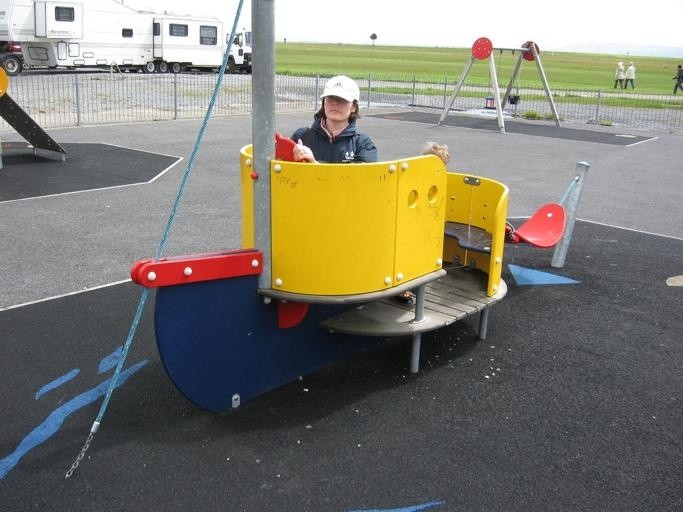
[483,51,524,110]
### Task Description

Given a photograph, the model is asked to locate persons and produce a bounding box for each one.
[612,61,624,89]
[671,64,682,95]
[420,140,450,165]
[289,75,413,305]
[623,61,634,90]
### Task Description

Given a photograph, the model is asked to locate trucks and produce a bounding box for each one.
[1,1,254,77]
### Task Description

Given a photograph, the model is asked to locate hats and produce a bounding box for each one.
[320,76,360,102]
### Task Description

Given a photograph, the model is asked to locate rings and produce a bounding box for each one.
[303,148,307,155]
